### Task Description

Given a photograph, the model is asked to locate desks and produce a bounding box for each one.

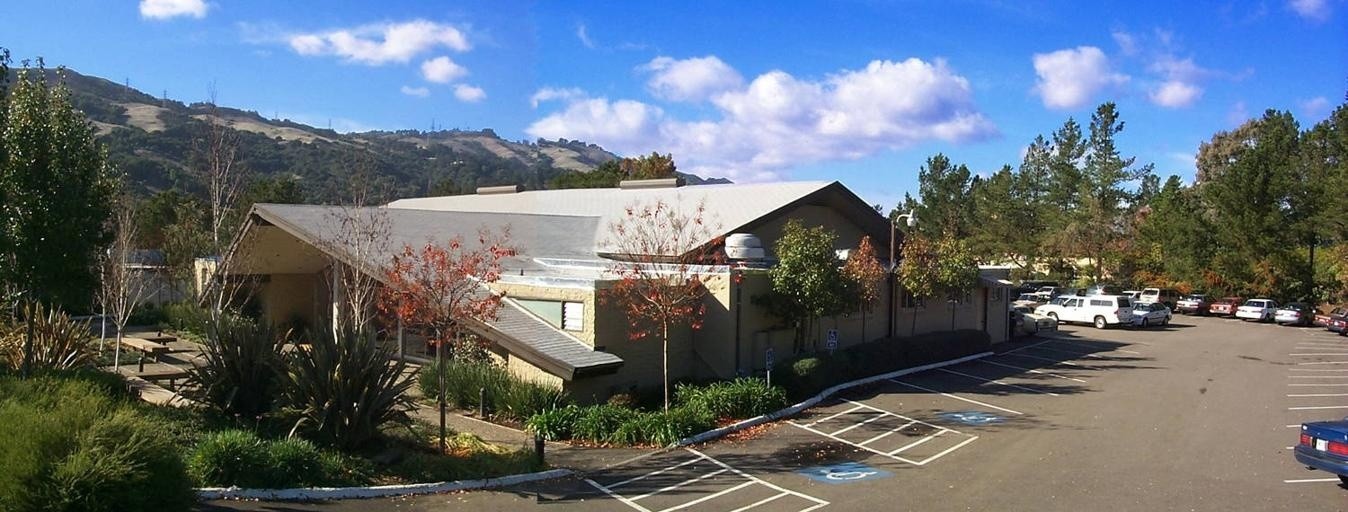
[98,329,196,406]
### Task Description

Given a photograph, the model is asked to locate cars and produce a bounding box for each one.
[1015,306,1058,333]
[1209,296,1245,318]
[1009,306,1025,332]
[1131,301,1174,329]
[1035,287,1064,303]
[1327,313,1348,334]
[1175,294,1218,316]
[1292,417,1348,490]
[1235,298,1280,322]
[1274,302,1324,327]
[1091,285,1142,300]
[1016,293,1050,313]
[1313,305,1347,327]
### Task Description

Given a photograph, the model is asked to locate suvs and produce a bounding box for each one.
[1138,287,1185,311]
[1035,295,1135,329]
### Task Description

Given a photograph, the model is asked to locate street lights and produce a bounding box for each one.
[888,206,921,343]
